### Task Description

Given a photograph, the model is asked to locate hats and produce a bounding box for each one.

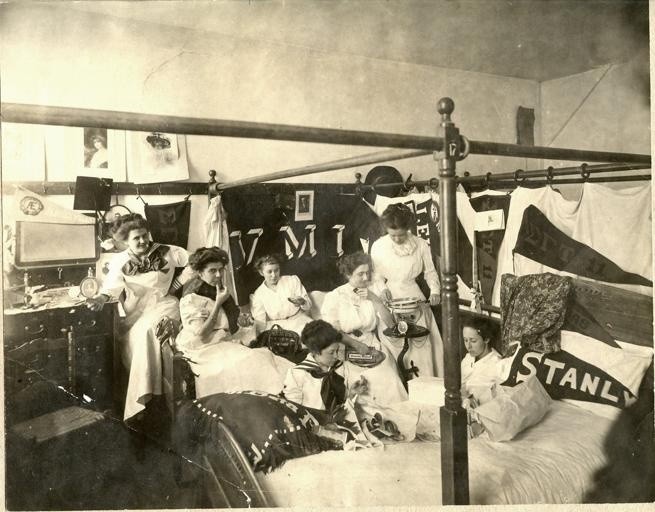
[365,165,403,205]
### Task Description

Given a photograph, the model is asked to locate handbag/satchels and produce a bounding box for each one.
[258,323,302,355]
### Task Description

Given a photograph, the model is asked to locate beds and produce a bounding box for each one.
[161,296,503,452]
[197,274,654,508]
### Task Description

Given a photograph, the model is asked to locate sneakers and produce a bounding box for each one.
[126,420,143,434]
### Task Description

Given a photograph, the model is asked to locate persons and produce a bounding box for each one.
[370,203,445,376]
[172,244,298,398]
[82,212,202,457]
[299,197,309,212]
[319,251,408,407]
[280,318,420,451]
[86,134,109,168]
[247,251,315,347]
[405,316,507,441]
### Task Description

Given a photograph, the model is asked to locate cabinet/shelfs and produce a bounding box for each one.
[5,303,117,417]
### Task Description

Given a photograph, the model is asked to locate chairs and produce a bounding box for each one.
[5,333,108,480]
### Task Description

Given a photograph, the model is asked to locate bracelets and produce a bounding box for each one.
[208,314,218,323]
